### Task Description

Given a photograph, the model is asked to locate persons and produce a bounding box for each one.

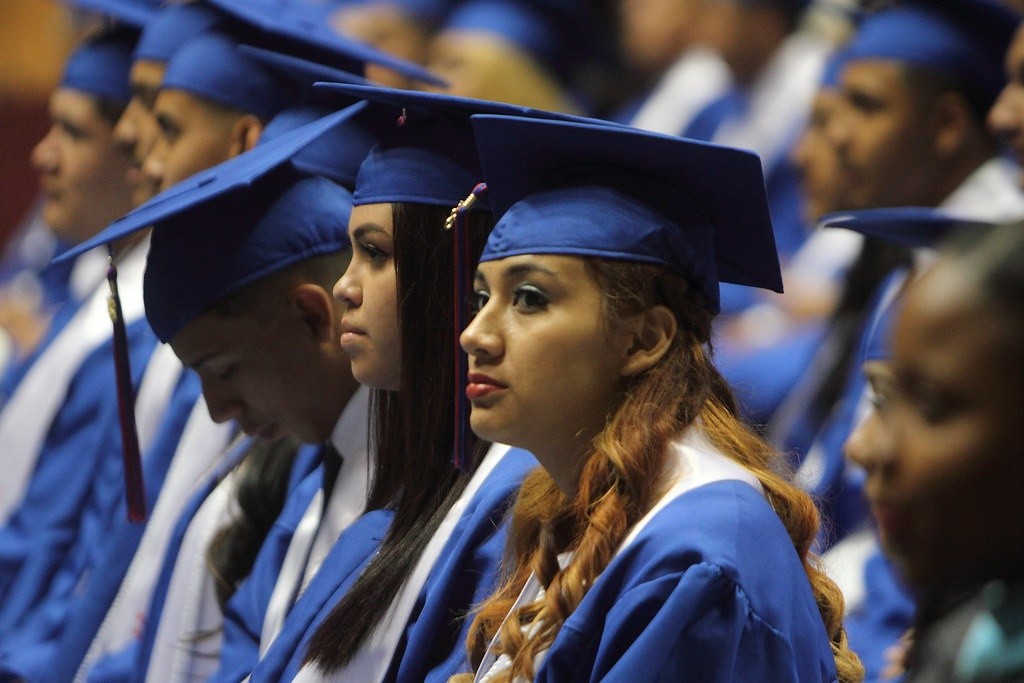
[451,111,863,683]
[0,2,1024,683]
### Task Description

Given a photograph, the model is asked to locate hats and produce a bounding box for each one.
[447,115,784,474]
[830,1,1024,107]
[53,0,165,105]
[131,0,224,62]
[434,2,569,67]
[229,45,393,187]
[52,97,367,530]
[312,81,629,217]
[824,210,1003,268]
[154,2,449,123]
